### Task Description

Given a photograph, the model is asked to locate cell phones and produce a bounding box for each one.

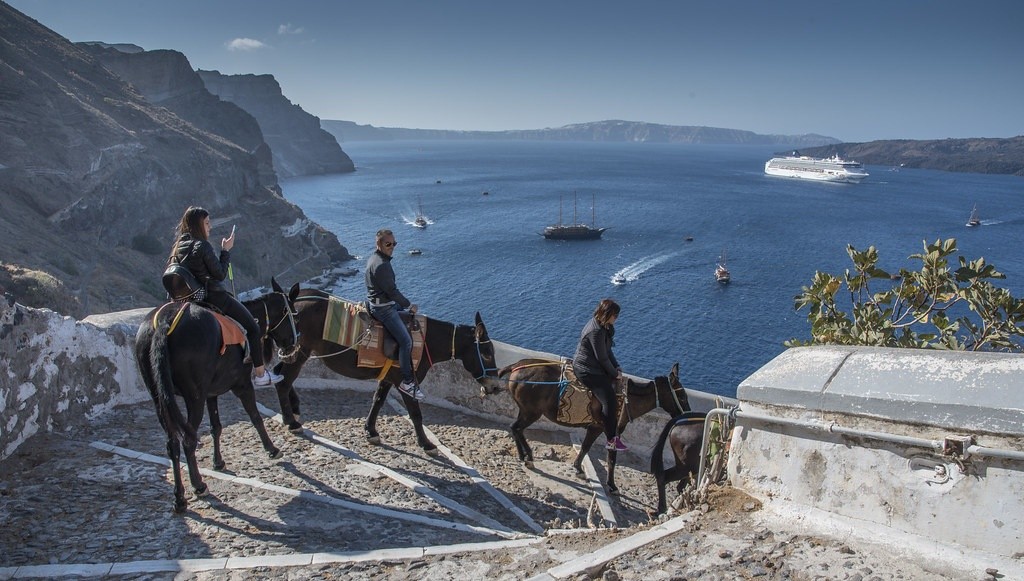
[231,225,235,237]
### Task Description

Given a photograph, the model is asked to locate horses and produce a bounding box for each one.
[135,275,301,512]
[650,411,707,516]
[499,358,691,495]
[273,288,499,455]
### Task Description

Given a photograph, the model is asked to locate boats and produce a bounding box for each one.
[416,217,426,226]
[410,251,423,254]
[764,151,870,184]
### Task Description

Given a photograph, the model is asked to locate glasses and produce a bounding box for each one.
[614,314,619,318]
[380,240,397,247]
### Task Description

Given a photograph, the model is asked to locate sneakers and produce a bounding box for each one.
[398,380,426,400]
[254,371,284,386]
[606,436,628,451]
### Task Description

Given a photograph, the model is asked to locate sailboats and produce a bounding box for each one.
[969,202,982,226]
[713,249,730,284]
[536,190,616,239]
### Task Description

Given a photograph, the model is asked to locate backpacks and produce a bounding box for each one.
[162,248,207,303]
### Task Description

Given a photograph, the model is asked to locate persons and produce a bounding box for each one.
[168,205,284,386]
[365,230,425,400]
[572,299,628,451]
